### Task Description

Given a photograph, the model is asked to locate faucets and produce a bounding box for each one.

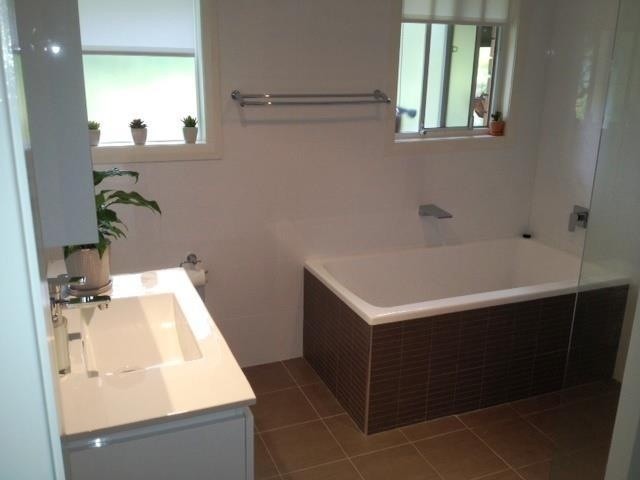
[567,210,588,232]
[51,275,113,312]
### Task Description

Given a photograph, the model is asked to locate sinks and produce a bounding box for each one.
[73,290,205,377]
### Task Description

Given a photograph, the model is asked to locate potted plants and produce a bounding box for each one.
[489,111,505,136]
[180,116,198,144]
[87,120,101,146]
[64,166,161,295]
[129,119,147,144]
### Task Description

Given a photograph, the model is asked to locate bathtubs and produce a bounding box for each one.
[302,234,631,329]
